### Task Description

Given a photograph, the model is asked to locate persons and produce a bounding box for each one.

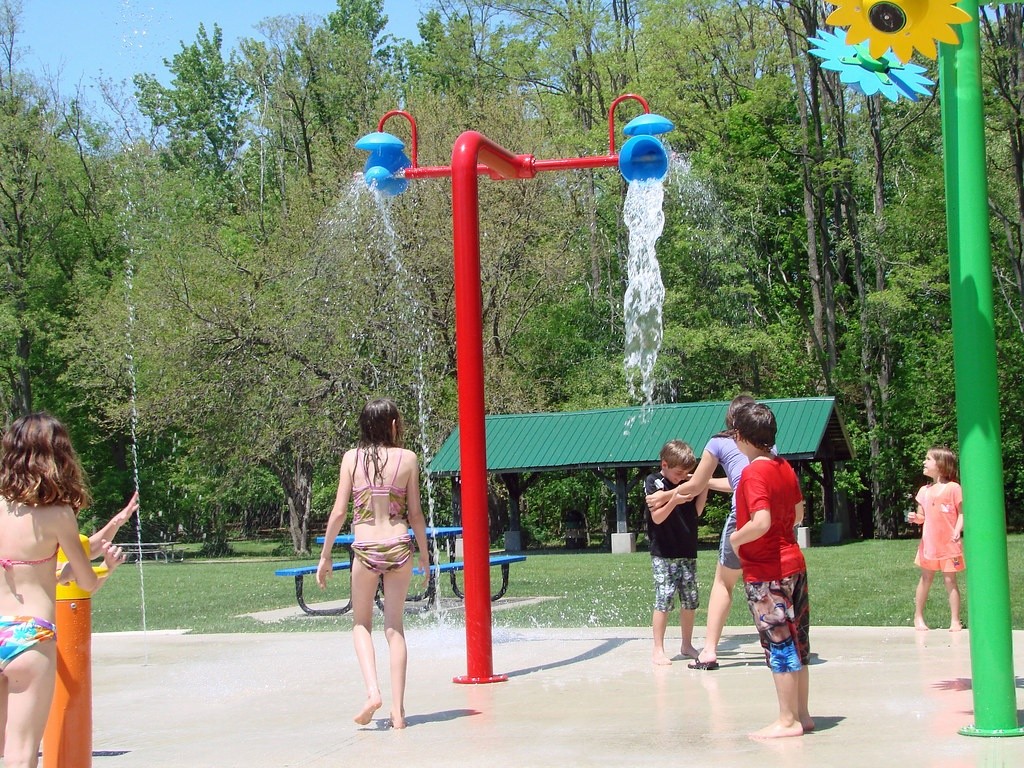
[906,448,968,633]
[642,438,711,666]
[311,394,430,728]
[0,411,100,768]
[643,395,781,672]
[729,403,815,739]
[88,489,140,600]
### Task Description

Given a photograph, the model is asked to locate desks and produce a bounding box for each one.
[111,541,182,564]
[314,526,464,618]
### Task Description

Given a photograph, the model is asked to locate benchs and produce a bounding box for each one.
[274,562,352,616]
[118,550,163,564]
[412,555,528,613]
[128,547,190,563]
